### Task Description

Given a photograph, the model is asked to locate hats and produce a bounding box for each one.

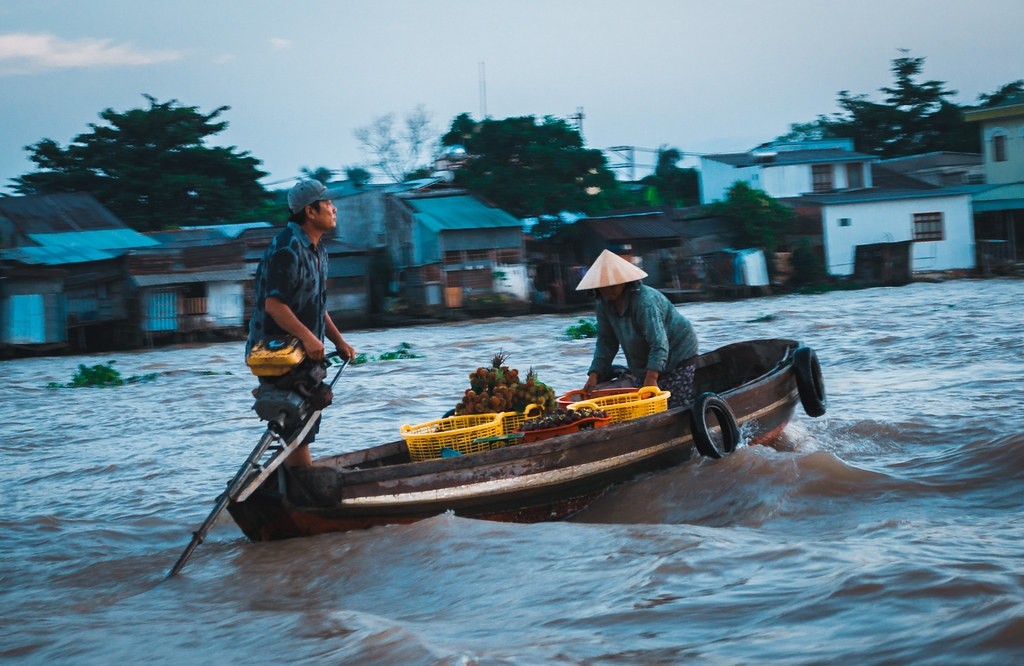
[288,180,344,214]
[575,249,648,290]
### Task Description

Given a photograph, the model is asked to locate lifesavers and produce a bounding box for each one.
[793,345,828,417]
[690,391,741,460]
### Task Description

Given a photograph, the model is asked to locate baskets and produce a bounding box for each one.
[502,404,545,446]
[555,386,671,423]
[400,412,504,461]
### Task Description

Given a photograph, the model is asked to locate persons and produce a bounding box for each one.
[576,249,698,409]
[244,179,355,464]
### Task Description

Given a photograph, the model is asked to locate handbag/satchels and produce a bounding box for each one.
[249,335,304,376]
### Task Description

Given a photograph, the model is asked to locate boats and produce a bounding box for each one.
[646,235,778,303]
[219,335,827,543]
